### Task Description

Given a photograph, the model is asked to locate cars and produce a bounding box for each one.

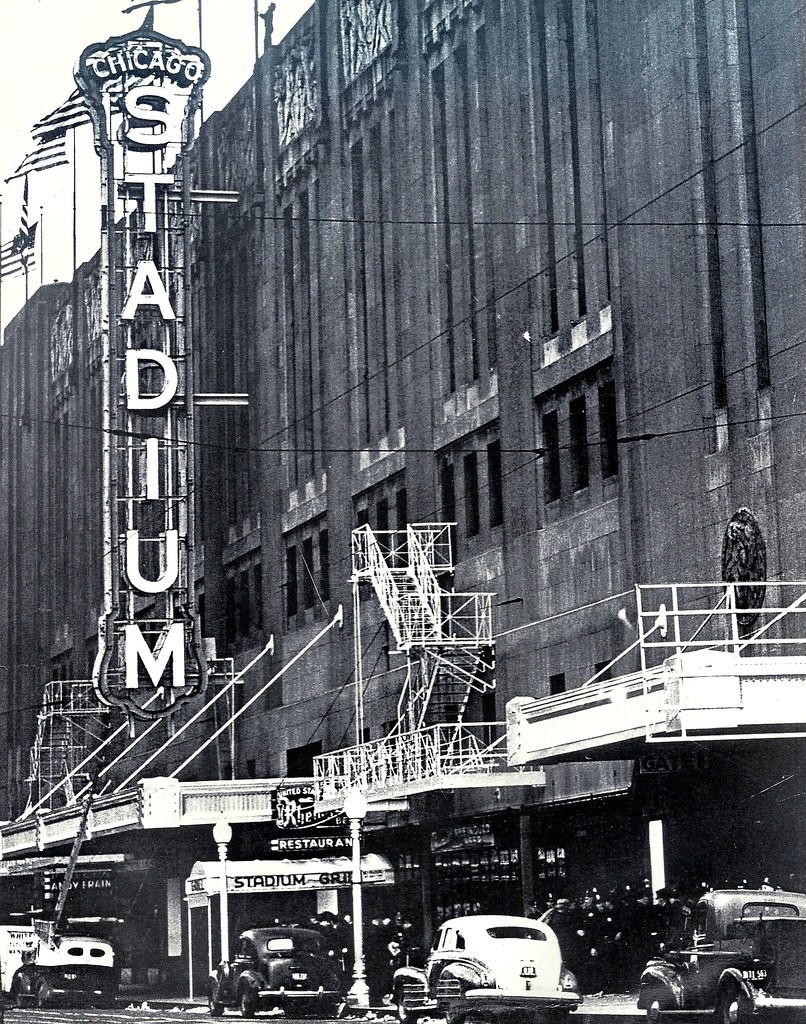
[208,928,343,1018]
[392,915,583,1024]
[637,888,806,1024]
[10,939,117,1008]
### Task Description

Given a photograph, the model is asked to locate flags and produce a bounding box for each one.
[2,133,70,184]
[28,88,120,141]
[0,222,38,282]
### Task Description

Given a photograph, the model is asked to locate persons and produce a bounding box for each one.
[279,911,412,986]
[548,877,698,992]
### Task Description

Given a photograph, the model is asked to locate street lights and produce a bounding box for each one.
[214,815,234,964]
[342,783,370,1017]
